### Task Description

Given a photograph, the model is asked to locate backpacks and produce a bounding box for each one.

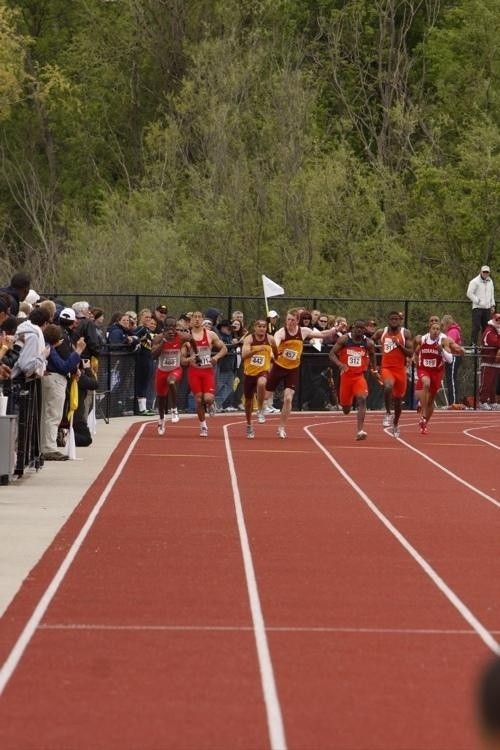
[464,396,480,409]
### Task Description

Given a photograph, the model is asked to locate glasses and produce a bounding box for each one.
[176,328,184,332]
[482,270,490,274]
[319,320,328,323]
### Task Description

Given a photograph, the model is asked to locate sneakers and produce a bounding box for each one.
[42,450,69,462]
[417,415,431,434]
[261,405,281,414]
[478,402,500,411]
[355,429,368,442]
[208,402,216,417]
[222,406,237,412]
[199,421,209,437]
[136,409,157,416]
[171,407,181,424]
[277,427,288,439]
[157,417,166,436]
[382,412,393,427]
[392,425,400,439]
[256,410,265,424]
[323,402,344,411]
[246,425,255,439]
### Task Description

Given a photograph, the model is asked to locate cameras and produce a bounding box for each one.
[80,359,88,366]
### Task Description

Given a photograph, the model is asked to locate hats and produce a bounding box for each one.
[366,320,377,327]
[60,306,78,321]
[480,264,491,273]
[492,313,500,321]
[156,303,169,311]
[267,309,279,319]
[74,309,88,319]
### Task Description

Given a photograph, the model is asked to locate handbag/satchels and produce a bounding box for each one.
[448,404,465,410]
